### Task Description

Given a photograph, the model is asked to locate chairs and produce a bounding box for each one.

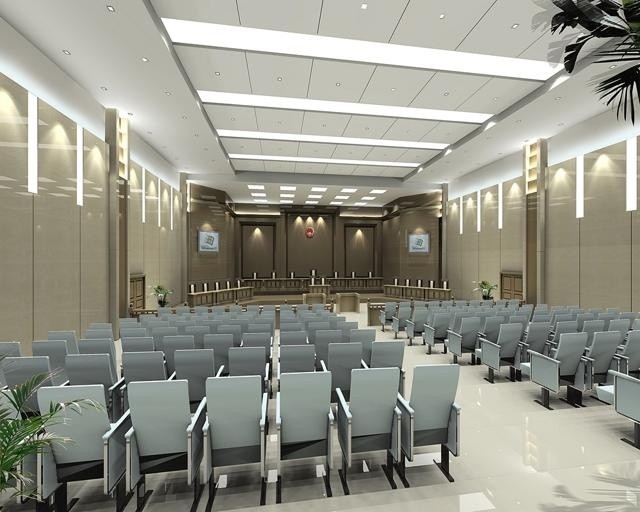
[189,270,449,293]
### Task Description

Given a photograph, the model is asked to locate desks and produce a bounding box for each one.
[188,287,254,307]
[384,285,451,299]
